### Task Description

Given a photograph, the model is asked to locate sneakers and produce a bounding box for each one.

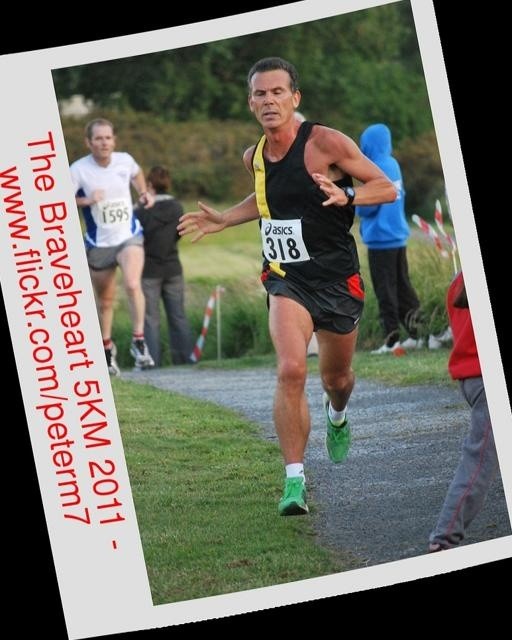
[278,476,310,514]
[400,336,425,351]
[324,394,351,465]
[105,344,122,376]
[371,340,401,355]
[129,337,155,369]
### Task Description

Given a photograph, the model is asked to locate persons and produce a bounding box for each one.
[417,273,494,553]
[177,56,400,519]
[65,116,158,376]
[129,163,192,366]
[350,120,425,359]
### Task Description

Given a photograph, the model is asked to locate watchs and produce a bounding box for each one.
[342,185,356,208]
